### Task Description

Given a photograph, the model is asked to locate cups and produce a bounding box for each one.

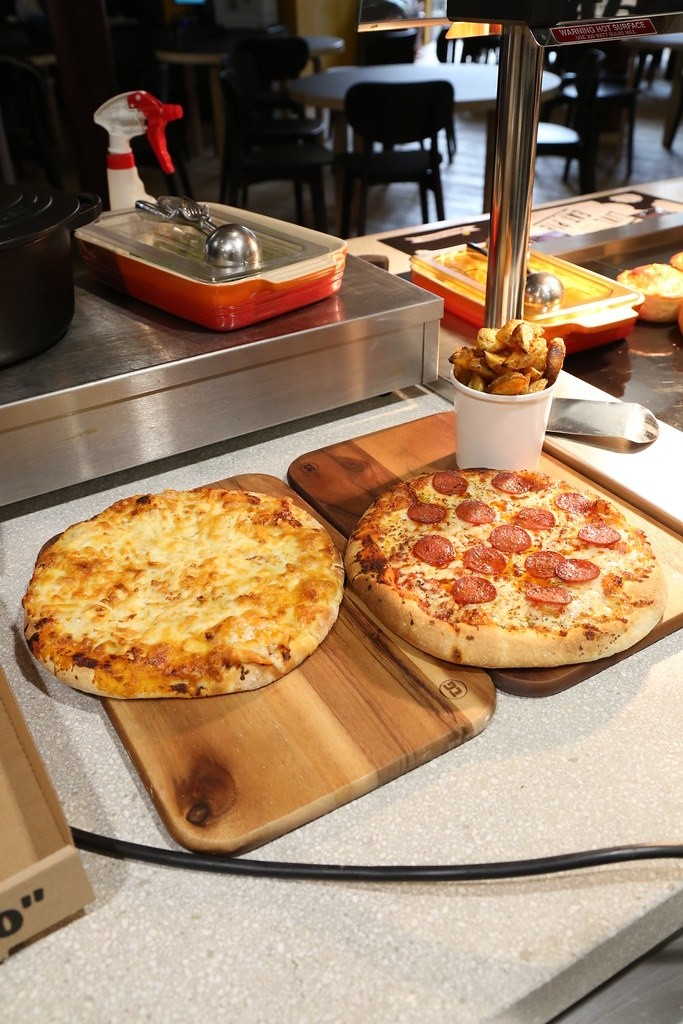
[448,363,559,469]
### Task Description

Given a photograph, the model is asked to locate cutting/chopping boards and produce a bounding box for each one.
[99,411,683,857]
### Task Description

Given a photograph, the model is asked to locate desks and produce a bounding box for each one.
[300,35,344,141]
[623,32,683,150]
[157,49,230,158]
[294,64,562,236]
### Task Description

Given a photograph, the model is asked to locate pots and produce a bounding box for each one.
[0,185,102,370]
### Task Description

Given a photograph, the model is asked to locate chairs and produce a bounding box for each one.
[332,80,456,239]
[218,36,336,233]
[437,0,636,195]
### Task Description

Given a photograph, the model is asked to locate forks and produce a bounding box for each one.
[184,209,217,234]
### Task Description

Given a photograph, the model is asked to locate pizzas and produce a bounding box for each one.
[344,466,666,667]
[23,489,343,701]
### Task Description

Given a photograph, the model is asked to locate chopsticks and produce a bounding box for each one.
[209,215,301,255]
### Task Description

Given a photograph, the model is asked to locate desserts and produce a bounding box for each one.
[616,253,683,334]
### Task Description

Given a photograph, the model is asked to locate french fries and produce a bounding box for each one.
[449,319,566,396]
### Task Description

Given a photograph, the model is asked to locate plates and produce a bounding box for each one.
[410,240,646,353]
[75,201,350,331]
